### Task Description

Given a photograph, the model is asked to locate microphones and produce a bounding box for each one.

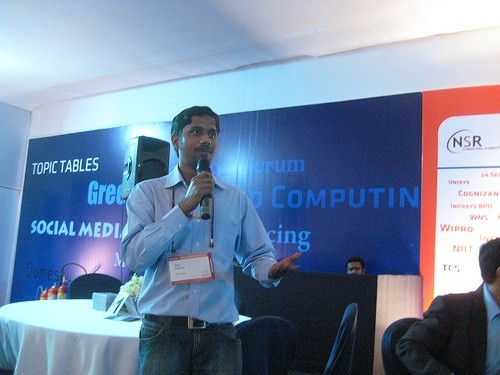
[196,159,211,220]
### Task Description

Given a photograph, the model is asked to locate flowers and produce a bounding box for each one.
[126,272,143,299]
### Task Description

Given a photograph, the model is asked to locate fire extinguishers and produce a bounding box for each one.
[39,274,67,300]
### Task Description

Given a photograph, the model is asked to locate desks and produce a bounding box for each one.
[0,299,252,375]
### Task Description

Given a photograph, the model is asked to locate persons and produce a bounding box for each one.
[118,106,301,375]
[346,255,365,276]
[395,237,500,375]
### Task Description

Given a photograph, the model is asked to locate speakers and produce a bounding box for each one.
[121,136,170,200]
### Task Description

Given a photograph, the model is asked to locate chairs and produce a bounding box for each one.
[236,316,297,375]
[381,318,423,375]
[69,274,123,299]
[321,303,358,375]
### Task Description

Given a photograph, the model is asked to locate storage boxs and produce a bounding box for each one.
[91,292,118,311]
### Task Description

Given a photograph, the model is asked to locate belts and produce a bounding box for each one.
[144,313,233,329]
